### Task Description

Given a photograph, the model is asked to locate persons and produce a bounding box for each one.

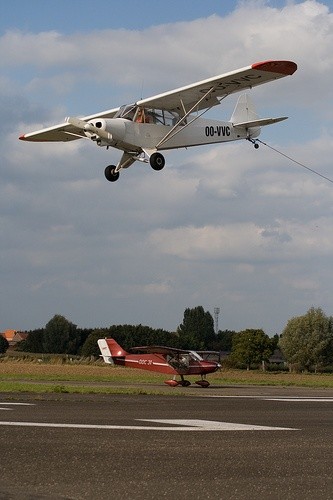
[135,108,149,123]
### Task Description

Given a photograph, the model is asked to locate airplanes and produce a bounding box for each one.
[17,59,298,182]
[97,337,223,387]
